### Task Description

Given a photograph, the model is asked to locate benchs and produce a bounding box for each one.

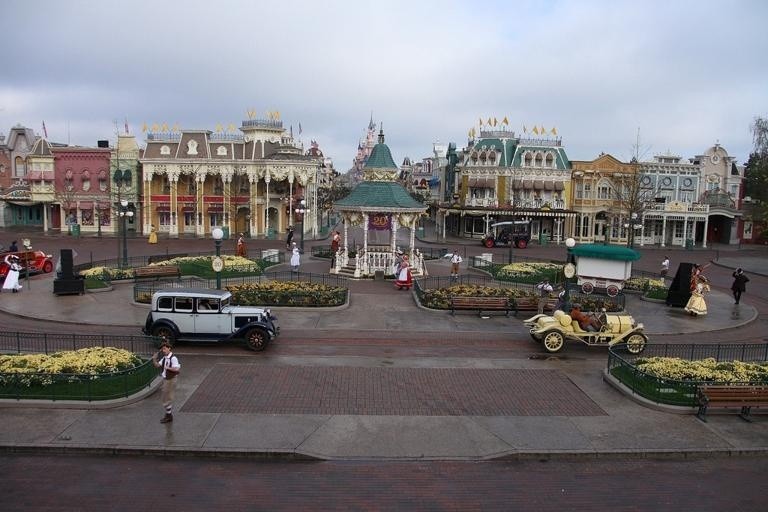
[450,296,559,317]
[694,384,768,422]
[132,265,182,283]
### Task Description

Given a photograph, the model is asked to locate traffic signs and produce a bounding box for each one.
[211,257,224,272]
[564,262,575,279]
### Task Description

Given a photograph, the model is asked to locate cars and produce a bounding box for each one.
[523,307,652,357]
[0,251,53,281]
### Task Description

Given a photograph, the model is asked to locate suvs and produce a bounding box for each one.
[142,287,282,353]
[480,219,532,249]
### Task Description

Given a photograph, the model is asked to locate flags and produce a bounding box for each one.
[465,115,558,143]
[142,109,282,135]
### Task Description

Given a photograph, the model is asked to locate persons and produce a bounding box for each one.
[555,284,570,312]
[394,252,405,280]
[9,240,19,252]
[290,242,301,272]
[571,303,605,333]
[535,278,554,314]
[151,342,181,425]
[147,223,158,245]
[236,231,248,258]
[690,263,711,296]
[286,224,294,250]
[659,256,670,282]
[730,267,750,307]
[330,229,341,253]
[2,253,24,293]
[449,250,463,283]
[395,255,415,291]
[684,274,711,316]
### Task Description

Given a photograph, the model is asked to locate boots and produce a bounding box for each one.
[160,414,172,423]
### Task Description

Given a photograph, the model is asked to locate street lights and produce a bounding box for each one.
[482,213,566,245]
[114,199,134,270]
[623,212,643,249]
[20,237,33,291]
[562,237,576,316]
[212,228,225,292]
